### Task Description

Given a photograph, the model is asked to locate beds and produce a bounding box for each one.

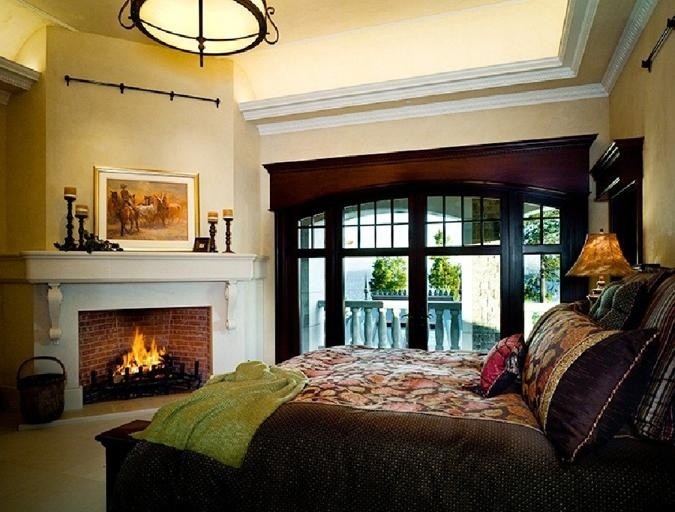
[95,266,675,512]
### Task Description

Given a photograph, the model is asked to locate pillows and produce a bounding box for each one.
[481,271,675,463]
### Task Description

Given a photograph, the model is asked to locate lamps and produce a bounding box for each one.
[118,0,279,67]
[566,228,634,295]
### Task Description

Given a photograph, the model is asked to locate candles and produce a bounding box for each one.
[64,187,76,196]
[208,212,218,219]
[76,205,89,215]
[223,209,233,217]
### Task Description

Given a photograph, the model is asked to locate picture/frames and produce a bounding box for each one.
[93,166,200,252]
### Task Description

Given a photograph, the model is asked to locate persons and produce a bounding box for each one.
[119,184,133,207]
